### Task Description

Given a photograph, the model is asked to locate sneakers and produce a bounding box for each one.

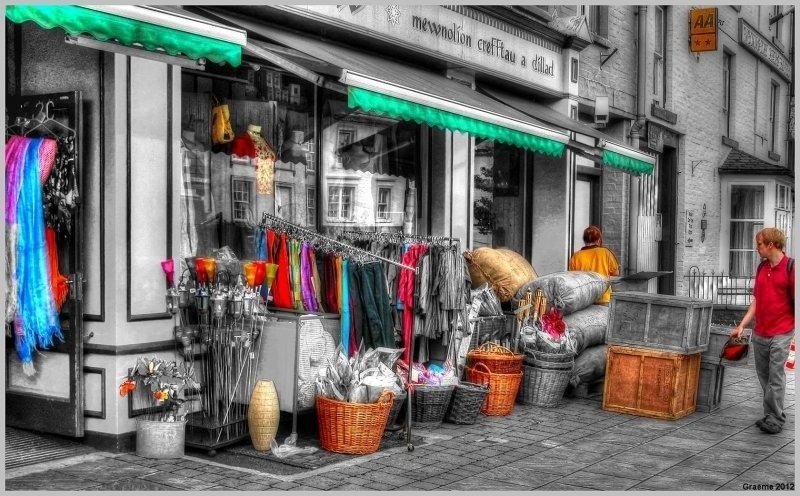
[755,415,784,434]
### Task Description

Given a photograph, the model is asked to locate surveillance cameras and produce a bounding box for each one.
[593,96,611,125]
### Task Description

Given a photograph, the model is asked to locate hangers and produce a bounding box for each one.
[340,225,461,253]
[255,213,384,268]
[4,100,76,144]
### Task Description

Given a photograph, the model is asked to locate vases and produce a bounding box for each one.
[247,377,281,452]
[132,409,189,461]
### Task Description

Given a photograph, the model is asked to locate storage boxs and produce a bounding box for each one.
[599,286,729,422]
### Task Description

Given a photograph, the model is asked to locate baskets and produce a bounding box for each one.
[313,389,395,457]
[384,341,579,432]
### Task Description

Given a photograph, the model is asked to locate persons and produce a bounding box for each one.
[727,227,795,436]
[231,124,276,162]
[341,144,375,172]
[568,224,621,308]
[280,130,307,163]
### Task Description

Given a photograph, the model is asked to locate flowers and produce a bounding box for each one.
[113,350,202,424]
